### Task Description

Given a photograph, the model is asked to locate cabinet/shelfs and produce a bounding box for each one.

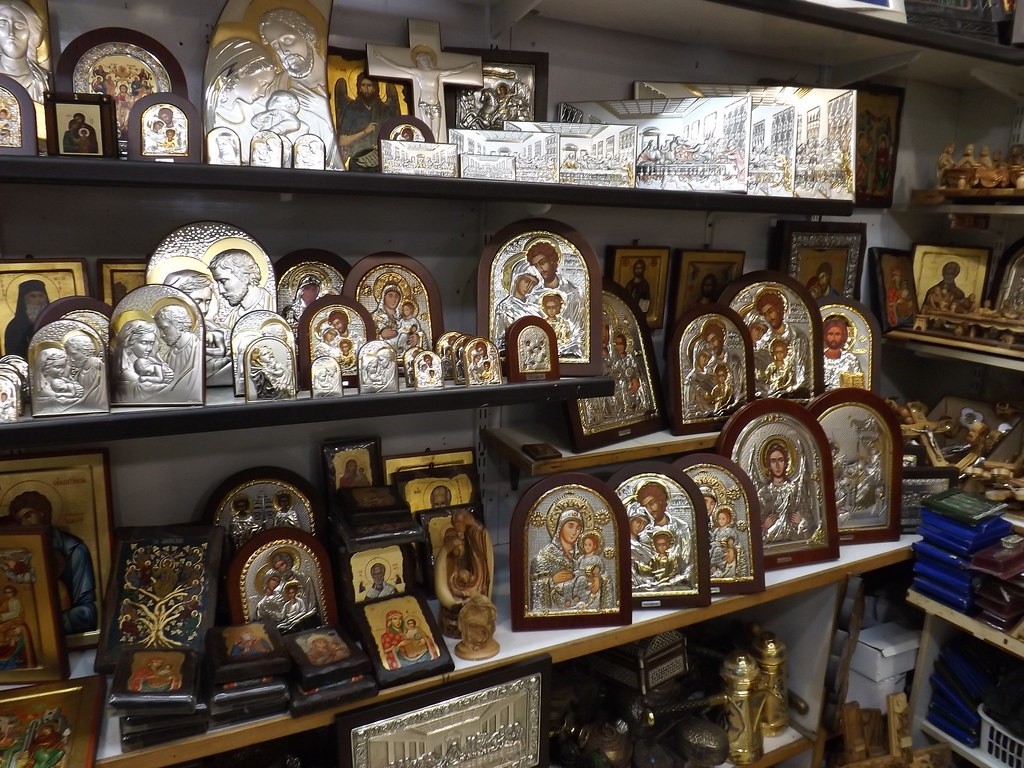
[0,156,912,768]
[898,198,1024,768]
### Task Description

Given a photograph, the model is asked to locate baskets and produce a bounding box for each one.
[977,703,1024,767]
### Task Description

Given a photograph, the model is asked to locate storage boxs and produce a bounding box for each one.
[839,621,921,714]
[975,702,1024,768]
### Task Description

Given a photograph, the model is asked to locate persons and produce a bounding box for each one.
[936,143,1024,180]
[596,290,811,420]
[32,305,202,415]
[493,241,585,359]
[627,481,694,593]
[10,491,98,636]
[227,488,320,631]
[163,249,274,385]
[530,508,606,612]
[203,7,344,171]
[0,0,49,104]
[373,50,478,143]
[696,419,885,579]
[820,319,862,391]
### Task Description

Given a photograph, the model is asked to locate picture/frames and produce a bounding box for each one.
[1,28,1024,768]
